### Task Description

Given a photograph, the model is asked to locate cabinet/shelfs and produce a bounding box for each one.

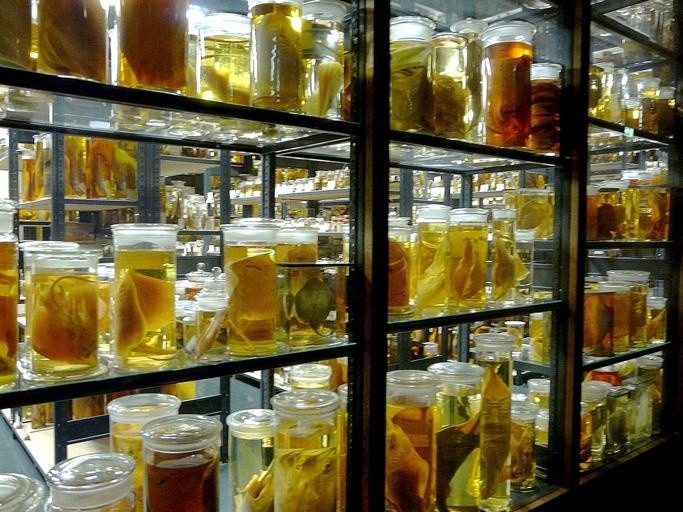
[1,0,680,512]
